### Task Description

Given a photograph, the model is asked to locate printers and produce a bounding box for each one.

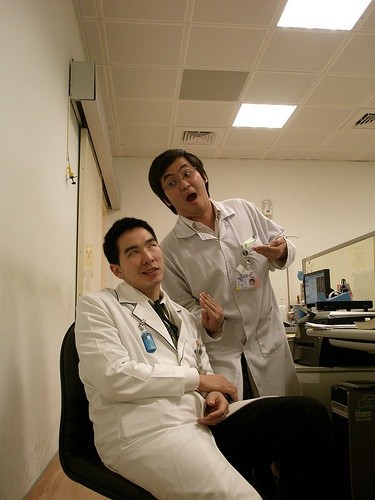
[292,310,375,368]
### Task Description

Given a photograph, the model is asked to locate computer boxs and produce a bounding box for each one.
[329,379,375,500]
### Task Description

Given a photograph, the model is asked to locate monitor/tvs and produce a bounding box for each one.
[303,269,330,307]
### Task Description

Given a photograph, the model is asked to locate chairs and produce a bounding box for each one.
[59,322,158,500]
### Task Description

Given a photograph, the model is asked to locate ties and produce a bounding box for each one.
[154,303,178,347]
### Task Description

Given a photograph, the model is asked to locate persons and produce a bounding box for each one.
[149,149,303,401]
[74,217,345,500]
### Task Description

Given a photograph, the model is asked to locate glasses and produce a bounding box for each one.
[163,167,196,191]
